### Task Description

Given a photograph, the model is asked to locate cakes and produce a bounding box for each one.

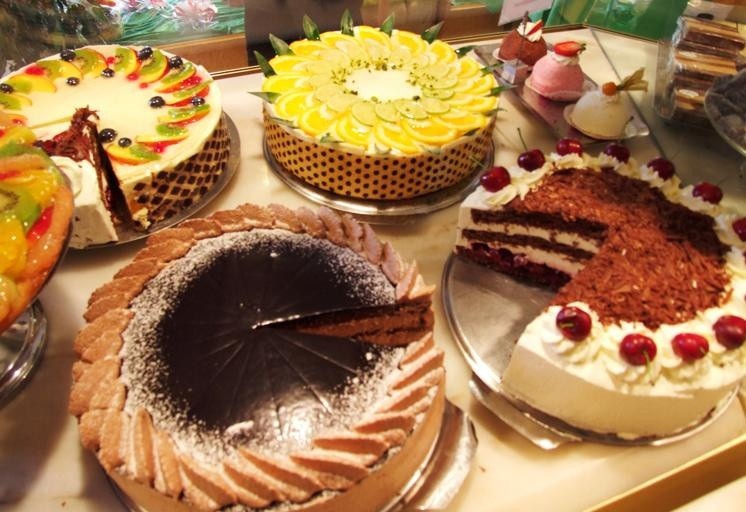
[1,42,233,249]
[66,202,447,511]
[449,118,746,442]
[496,9,646,139]
[248,8,518,202]
[1,139,73,332]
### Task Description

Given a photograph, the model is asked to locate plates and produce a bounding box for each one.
[440,244,742,452]
[63,95,240,255]
[471,40,651,148]
[109,399,479,509]
[262,138,498,218]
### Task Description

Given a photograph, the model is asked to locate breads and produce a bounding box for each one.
[659,0,746,153]
